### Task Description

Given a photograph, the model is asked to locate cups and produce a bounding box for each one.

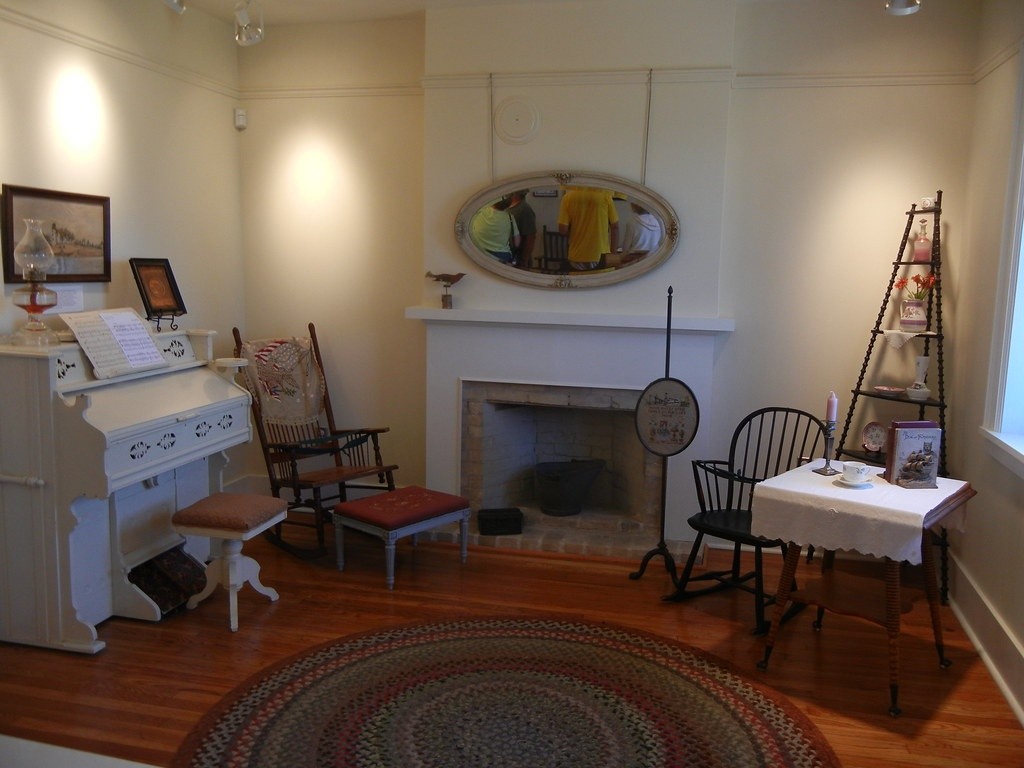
[843,462,871,482]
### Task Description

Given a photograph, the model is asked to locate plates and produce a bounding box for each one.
[863,422,888,451]
[835,474,875,486]
[874,386,905,396]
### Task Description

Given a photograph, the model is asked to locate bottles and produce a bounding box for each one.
[905,356,932,401]
[914,219,932,261]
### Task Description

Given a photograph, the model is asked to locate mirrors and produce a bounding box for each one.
[450,168,679,289]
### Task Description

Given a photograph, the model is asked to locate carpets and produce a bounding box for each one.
[169,613,845,768]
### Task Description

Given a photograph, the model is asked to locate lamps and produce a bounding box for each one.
[884,0,920,15]
[231,1,263,46]
[5,219,60,347]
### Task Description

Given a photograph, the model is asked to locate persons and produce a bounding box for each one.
[558,189,619,271]
[621,202,661,264]
[505,188,536,270]
[472,195,520,266]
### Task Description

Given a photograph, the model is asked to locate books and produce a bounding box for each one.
[884,419,941,485]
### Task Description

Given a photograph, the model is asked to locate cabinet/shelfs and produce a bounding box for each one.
[807,189,950,601]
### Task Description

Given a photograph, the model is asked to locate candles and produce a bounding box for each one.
[828,390,837,422]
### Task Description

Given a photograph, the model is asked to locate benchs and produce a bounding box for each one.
[332,485,473,589]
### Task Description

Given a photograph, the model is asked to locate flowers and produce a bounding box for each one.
[896,272,936,301]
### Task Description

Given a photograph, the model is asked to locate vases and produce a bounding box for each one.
[900,300,928,333]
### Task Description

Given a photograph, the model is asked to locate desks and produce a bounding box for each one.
[749,456,975,717]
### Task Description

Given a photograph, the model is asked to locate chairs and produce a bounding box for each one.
[663,405,826,634]
[543,224,572,275]
[232,322,397,567]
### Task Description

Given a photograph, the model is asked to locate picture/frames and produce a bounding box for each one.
[127,256,188,316]
[3,182,111,280]
[533,189,556,197]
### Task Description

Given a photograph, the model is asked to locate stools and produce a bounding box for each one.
[171,490,292,631]
